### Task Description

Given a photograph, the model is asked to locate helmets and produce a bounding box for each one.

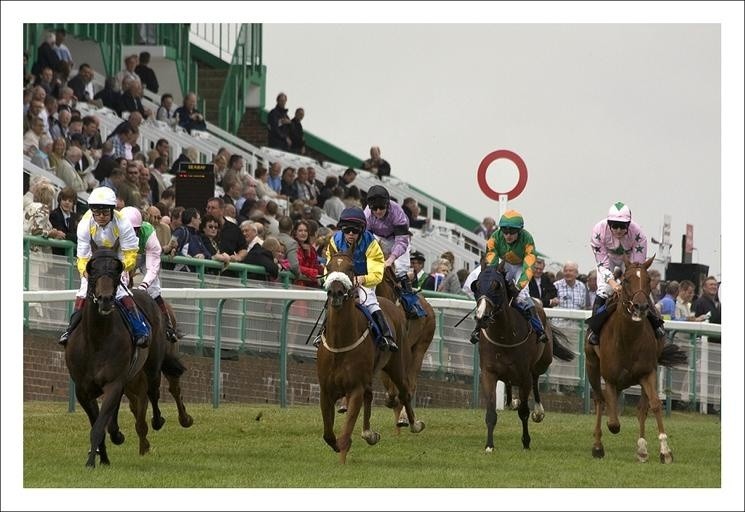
[366,185,391,208]
[607,202,631,227]
[87,187,117,208]
[499,210,524,234]
[337,207,367,232]
[120,206,142,227]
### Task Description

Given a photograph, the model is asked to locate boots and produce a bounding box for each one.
[395,279,427,319]
[526,306,549,343]
[154,295,181,342]
[60,297,85,343]
[371,310,399,352]
[120,296,149,348]
[654,324,665,339]
[588,295,605,345]
[470,328,480,343]
[316,315,326,348]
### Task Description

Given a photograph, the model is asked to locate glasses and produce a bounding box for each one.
[91,208,111,216]
[208,224,219,230]
[342,226,362,234]
[612,224,627,229]
[369,205,387,211]
[501,226,519,235]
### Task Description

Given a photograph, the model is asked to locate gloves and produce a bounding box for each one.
[509,287,519,299]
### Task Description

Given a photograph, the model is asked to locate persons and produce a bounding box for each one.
[530,259,597,396]
[586,201,666,345]
[362,146,390,181]
[364,185,428,320]
[646,269,721,415]
[312,206,399,351]
[389,196,500,374]
[468,208,548,344]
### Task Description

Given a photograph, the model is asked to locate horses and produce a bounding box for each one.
[65,234,193,469]
[315,232,437,467]
[474,256,577,453]
[584,249,689,465]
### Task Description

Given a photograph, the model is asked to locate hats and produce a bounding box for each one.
[410,249,425,261]
